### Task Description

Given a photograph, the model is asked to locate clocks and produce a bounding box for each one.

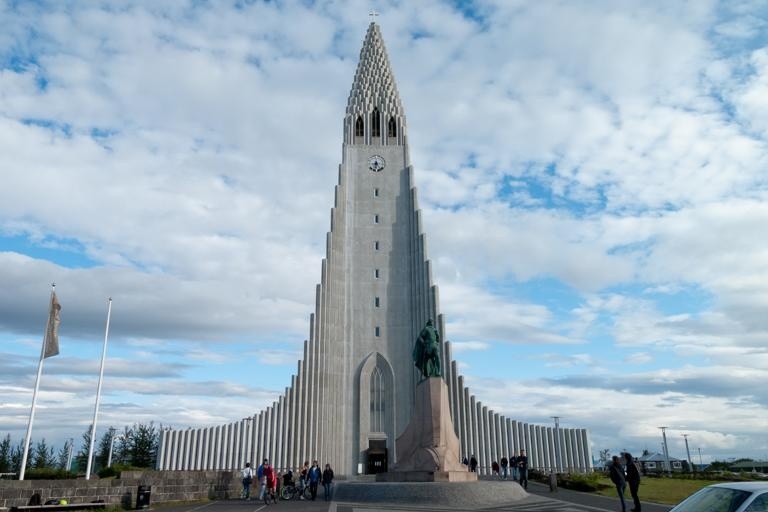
[367,155,386,172]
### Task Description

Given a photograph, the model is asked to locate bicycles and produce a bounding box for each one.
[281,477,313,500]
[264,478,279,505]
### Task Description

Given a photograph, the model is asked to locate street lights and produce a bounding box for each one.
[107,428,120,468]
[657,425,673,478]
[550,416,566,472]
[679,433,695,478]
[65,438,75,471]
[698,448,705,471]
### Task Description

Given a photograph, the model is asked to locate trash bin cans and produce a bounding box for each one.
[136,484,151,509]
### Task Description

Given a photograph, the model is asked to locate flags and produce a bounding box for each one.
[40,292,61,359]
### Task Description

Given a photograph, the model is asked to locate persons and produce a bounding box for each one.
[625,451,644,511]
[241,459,335,503]
[412,317,444,378]
[462,449,529,490]
[609,455,627,512]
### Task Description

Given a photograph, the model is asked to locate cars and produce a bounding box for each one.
[662,480,768,512]
